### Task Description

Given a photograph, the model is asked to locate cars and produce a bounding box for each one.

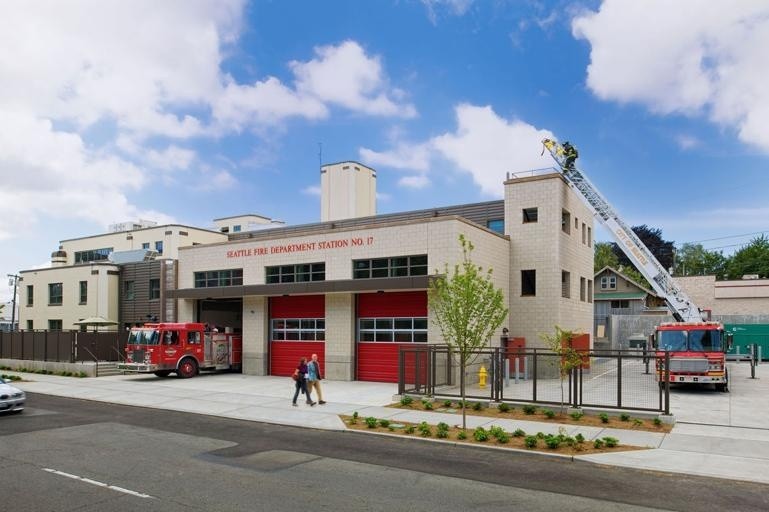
[0,373,29,418]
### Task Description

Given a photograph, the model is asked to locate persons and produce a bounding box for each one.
[292,358,317,407]
[307,353,326,404]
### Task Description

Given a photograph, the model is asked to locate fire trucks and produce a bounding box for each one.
[123,320,242,379]
[540,133,735,395]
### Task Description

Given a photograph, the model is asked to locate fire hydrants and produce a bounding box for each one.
[476,365,489,391]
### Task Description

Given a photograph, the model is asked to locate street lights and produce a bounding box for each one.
[6,272,26,330]
